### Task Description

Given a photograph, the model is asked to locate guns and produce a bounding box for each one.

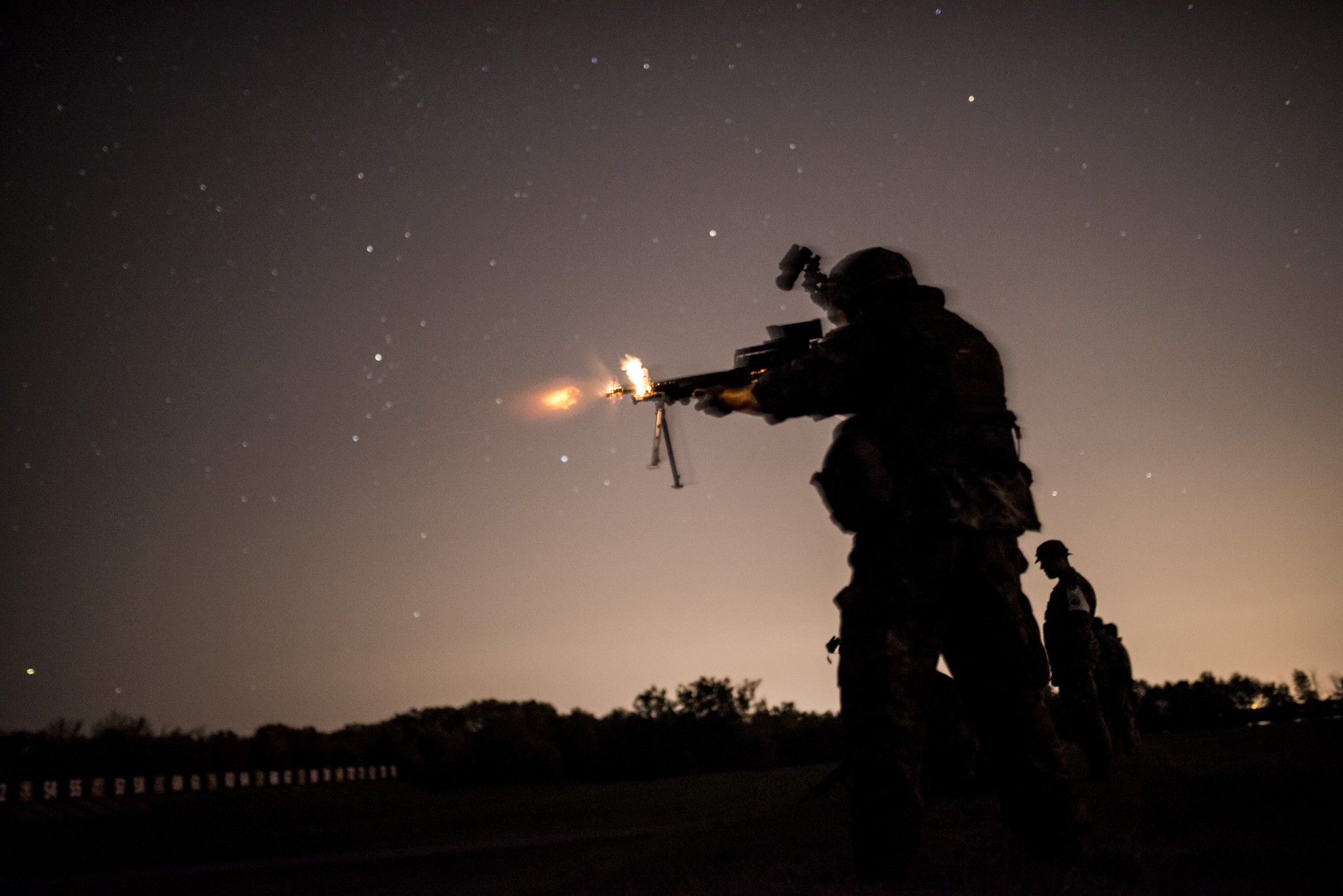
[604,318,824,489]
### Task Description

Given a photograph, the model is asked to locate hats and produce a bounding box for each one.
[1034,540,1072,564]
[810,245,947,311]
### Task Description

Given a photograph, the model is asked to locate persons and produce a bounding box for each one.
[694,249,1053,736]
[1034,540,1095,689]
